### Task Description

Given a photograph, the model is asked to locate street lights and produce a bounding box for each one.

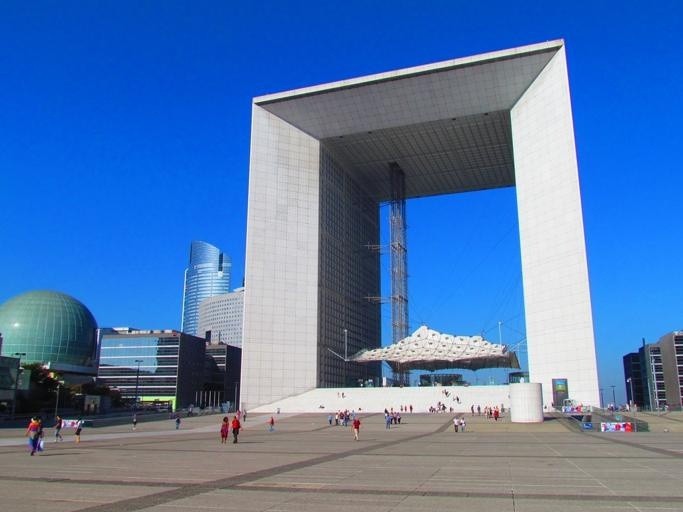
[627,377,637,432]
[133,358,144,416]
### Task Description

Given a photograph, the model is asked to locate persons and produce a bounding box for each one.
[218,417,229,444]
[428,389,460,413]
[34,416,45,452]
[452,416,459,433]
[268,416,274,429]
[230,416,240,443]
[469,404,499,422]
[70,417,82,443]
[131,416,137,432]
[51,414,64,443]
[173,416,181,430]
[458,417,466,434]
[241,409,247,422]
[235,409,240,422]
[325,409,358,428]
[350,416,360,441]
[382,404,413,434]
[22,415,40,457]
